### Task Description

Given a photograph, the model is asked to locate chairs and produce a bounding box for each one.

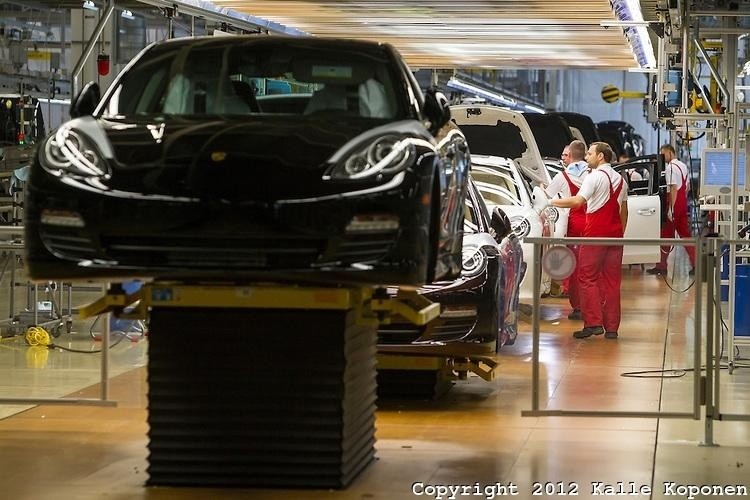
[166,81,389,116]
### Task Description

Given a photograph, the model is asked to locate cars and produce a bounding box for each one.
[22,27,673,356]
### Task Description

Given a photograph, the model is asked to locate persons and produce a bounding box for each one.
[558,144,571,296]
[646,145,696,274]
[619,154,642,183]
[537,139,595,321]
[536,141,628,339]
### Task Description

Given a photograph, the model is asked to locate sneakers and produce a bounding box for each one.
[605,331,618,338]
[568,309,582,320]
[574,326,604,338]
[646,268,667,275]
